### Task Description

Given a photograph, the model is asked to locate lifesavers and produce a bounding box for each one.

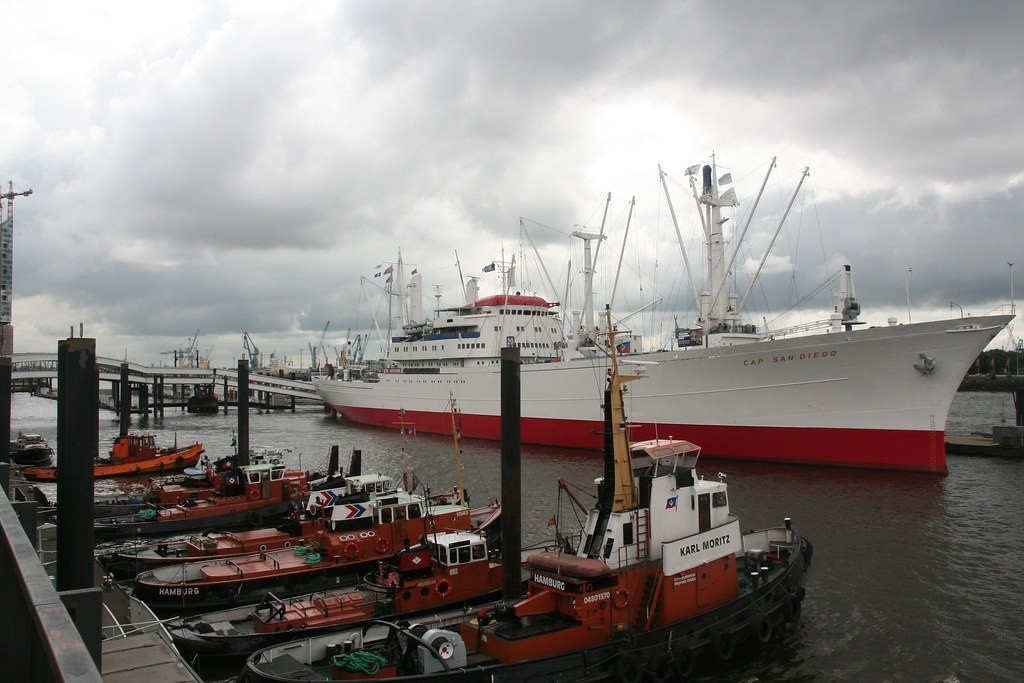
[613,588,631,608]
[345,543,359,559]
[250,489,260,500]
[375,538,389,553]
[435,579,452,598]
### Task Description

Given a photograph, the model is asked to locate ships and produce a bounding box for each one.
[310,155,1024,478]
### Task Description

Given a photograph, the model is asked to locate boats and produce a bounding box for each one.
[169,319,387,409]
[234,302,817,683]
[186,383,218,414]
[6,431,54,467]
[24,430,529,621]
[168,391,605,669]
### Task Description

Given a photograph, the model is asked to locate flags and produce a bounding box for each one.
[385,276,394,283]
[684,165,700,176]
[666,496,677,509]
[547,517,556,528]
[717,172,733,186]
[383,267,393,275]
[222,460,233,469]
[410,268,418,275]
[405,427,416,437]
[374,272,381,277]
[225,475,239,486]
[374,264,381,270]
[505,266,516,287]
[616,341,629,354]
[718,187,737,206]
